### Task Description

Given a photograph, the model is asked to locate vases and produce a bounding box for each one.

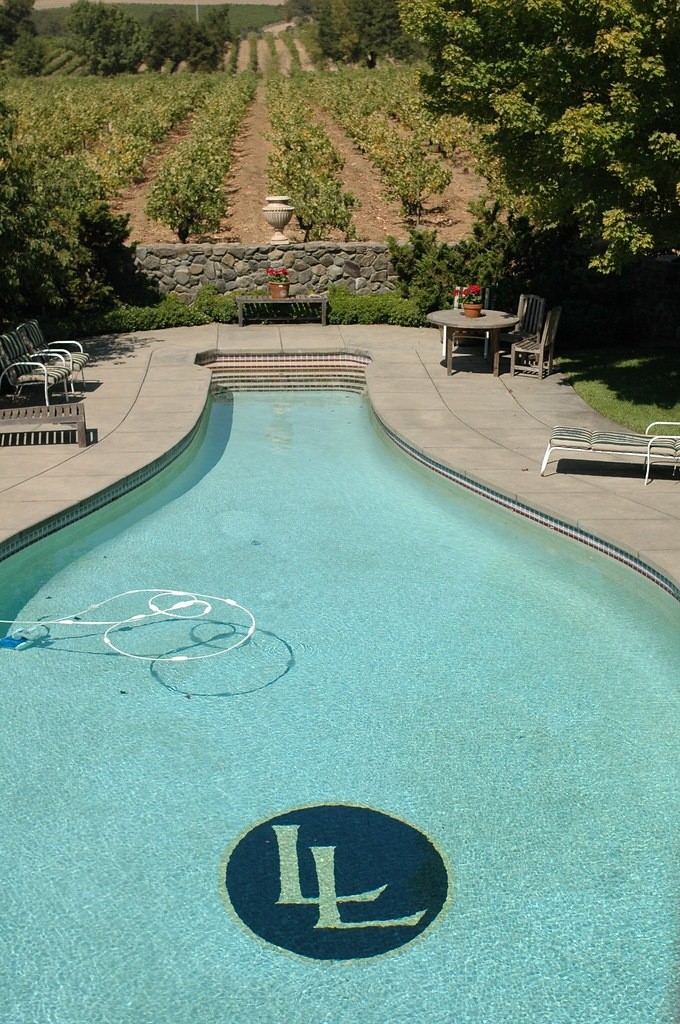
[269,281,290,298]
[463,303,482,318]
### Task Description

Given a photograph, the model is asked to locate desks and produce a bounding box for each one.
[426,309,520,377]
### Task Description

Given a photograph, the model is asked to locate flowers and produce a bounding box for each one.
[452,285,483,304]
[267,267,289,282]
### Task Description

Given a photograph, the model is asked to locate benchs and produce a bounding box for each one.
[236,295,328,327]
[0,403,86,448]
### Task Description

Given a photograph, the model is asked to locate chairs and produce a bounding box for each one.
[0,330,71,406]
[510,306,563,381]
[442,287,490,359]
[491,292,545,366]
[541,421,680,486]
[16,319,90,398]
